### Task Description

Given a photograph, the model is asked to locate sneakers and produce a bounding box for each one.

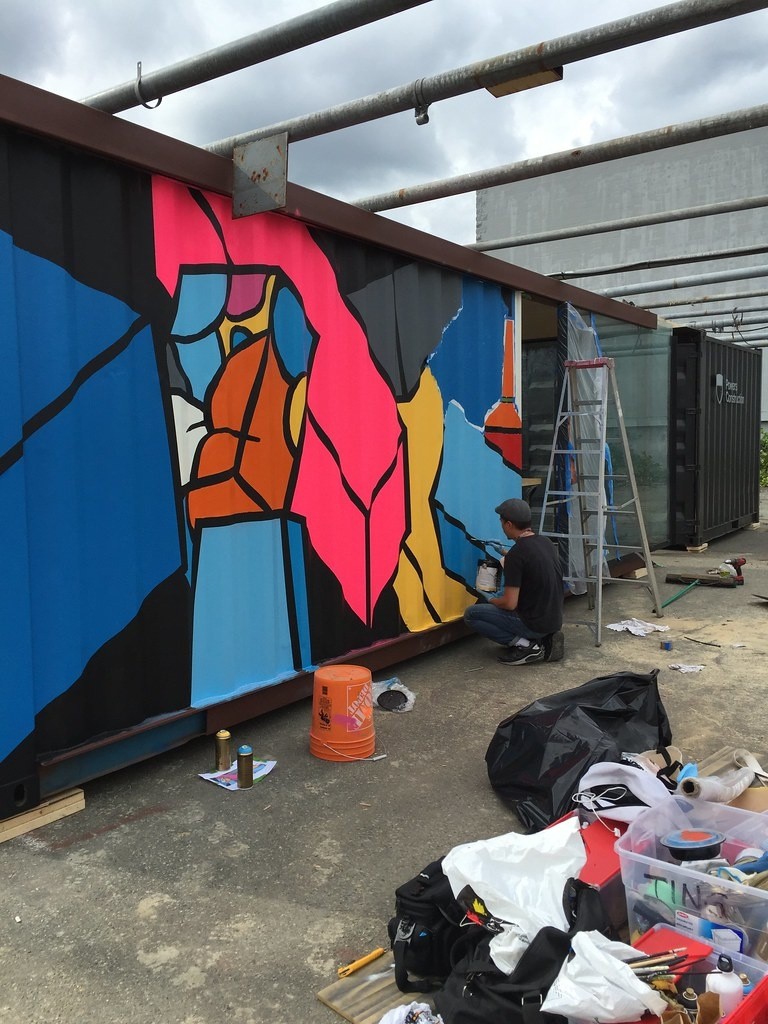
[545,631,564,661]
[499,642,546,665]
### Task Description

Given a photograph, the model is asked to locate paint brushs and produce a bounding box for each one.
[470,538,512,547]
[622,948,722,976]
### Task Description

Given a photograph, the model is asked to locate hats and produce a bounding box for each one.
[495,498,532,522]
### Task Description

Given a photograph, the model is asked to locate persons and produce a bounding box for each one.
[462,498,565,666]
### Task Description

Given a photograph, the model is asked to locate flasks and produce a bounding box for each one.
[705,953,743,1022]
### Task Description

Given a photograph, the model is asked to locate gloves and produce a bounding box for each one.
[483,538,505,553]
[478,589,497,603]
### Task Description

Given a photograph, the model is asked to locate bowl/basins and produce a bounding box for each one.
[660,830,726,860]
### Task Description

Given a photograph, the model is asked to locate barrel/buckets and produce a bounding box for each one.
[476,559,502,594]
[309,666,387,762]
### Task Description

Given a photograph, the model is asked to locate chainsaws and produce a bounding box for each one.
[718,558,746,585]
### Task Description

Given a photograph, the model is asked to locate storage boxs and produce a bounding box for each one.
[623,923,768,1024]
[615,794,768,961]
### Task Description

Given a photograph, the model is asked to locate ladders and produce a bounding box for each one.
[538,357,664,647]
